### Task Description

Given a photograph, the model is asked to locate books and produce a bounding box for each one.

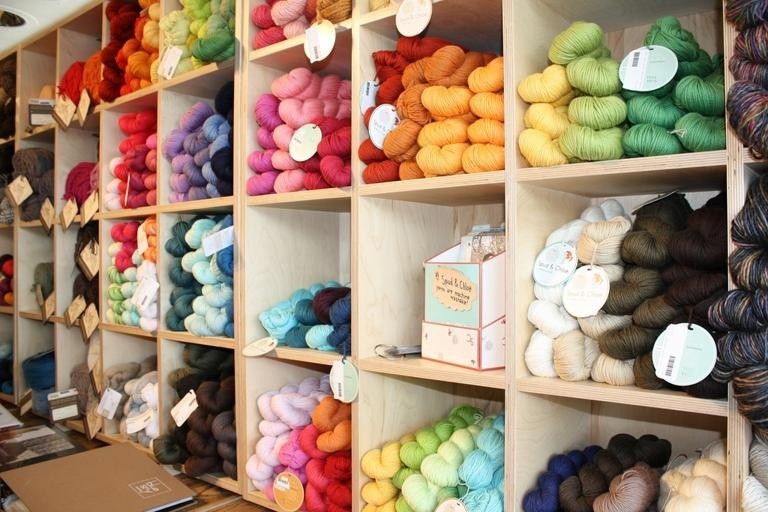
[0,404,198,512]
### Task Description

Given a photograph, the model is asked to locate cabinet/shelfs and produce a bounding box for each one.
[0,0,244,498]
[234,0,767,512]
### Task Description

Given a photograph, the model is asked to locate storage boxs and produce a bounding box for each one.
[419,233,507,374]
